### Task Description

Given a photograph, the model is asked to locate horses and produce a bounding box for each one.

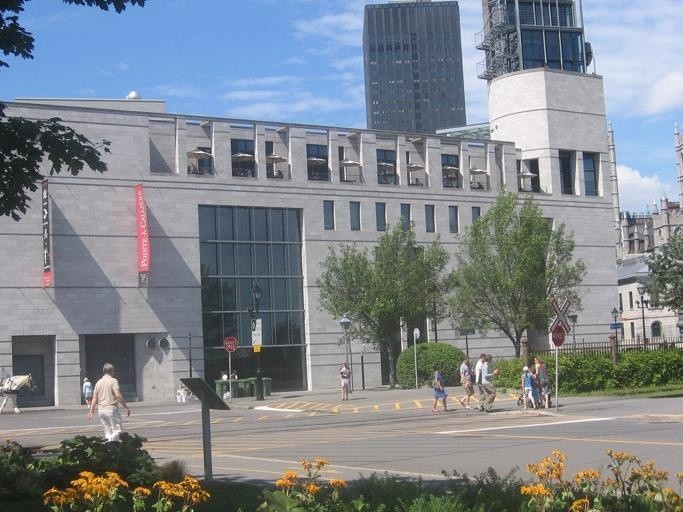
[0,372,38,414]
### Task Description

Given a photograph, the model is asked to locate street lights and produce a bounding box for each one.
[611,284,646,351]
[339,314,350,363]
[248,283,264,401]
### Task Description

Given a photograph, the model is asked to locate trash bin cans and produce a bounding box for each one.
[214,377,272,399]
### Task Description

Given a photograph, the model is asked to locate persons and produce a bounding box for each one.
[339,361,350,401]
[531,368,539,407]
[230,370,237,380]
[220,370,227,381]
[457,356,474,409]
[521,365,536,410]
[534,355,551,410]
[81,376,93,409]
[86,362,130,443]
[474,353,485,411]
[480,354,500,413]
[430,362,449,412]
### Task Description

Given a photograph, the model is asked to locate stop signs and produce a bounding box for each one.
[224,337,238,352]
[552,326,565,346]
